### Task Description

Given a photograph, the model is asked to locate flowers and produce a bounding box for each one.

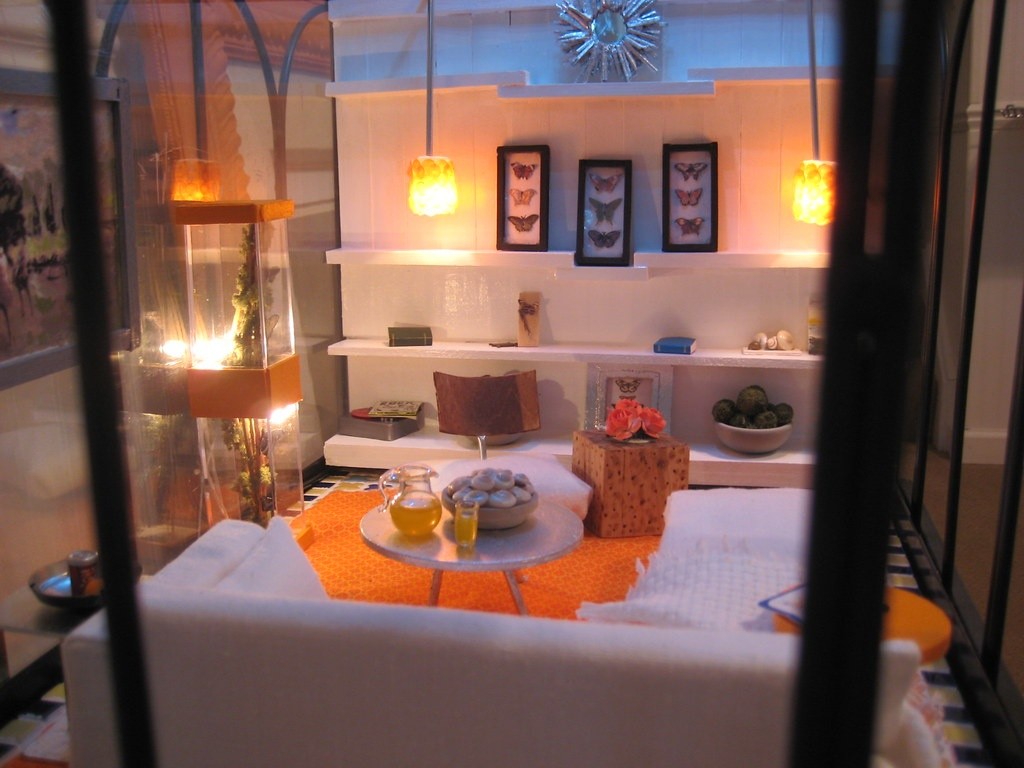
[605,398,668,439]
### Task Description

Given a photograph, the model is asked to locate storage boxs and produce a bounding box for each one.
[336,415,422,442]
[170,199,309,529]
[574,429,691,540]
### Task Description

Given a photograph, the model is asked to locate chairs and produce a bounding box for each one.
[431,368,543,459]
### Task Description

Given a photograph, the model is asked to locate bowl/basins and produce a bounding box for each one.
[441,481,539,531]
[714,419,793,455]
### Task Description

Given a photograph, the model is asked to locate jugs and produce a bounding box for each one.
[376,464,443,538]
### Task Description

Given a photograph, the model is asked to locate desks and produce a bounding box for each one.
[59,487,913,768]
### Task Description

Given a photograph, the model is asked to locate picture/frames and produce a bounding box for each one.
[661,141,720,253]
[496,143,550,252]
[584,362,673,436]
[0,67,143,390]
[573,158,631,268]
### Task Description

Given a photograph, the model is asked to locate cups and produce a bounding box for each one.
[452,500,478,548]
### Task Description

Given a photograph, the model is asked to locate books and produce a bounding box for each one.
[653,337,697,354]
[388,327,433,347]
[368,400,424,419]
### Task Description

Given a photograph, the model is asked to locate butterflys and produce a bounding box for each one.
[674,161,707,181]
[674,217,704,236]
[674,187,703,206]
[507,188,537,205]
[506,214,539,232]
[588,230,621,247]
[511,162,538,180]
[588,196,623,226]
[589,172,622,194]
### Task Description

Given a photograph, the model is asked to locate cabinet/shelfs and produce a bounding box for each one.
[322,247,827,490]
[927,126,1024,465]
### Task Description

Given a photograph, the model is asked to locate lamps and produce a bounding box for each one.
[407,0,460,218]
[789,0,836,228]
